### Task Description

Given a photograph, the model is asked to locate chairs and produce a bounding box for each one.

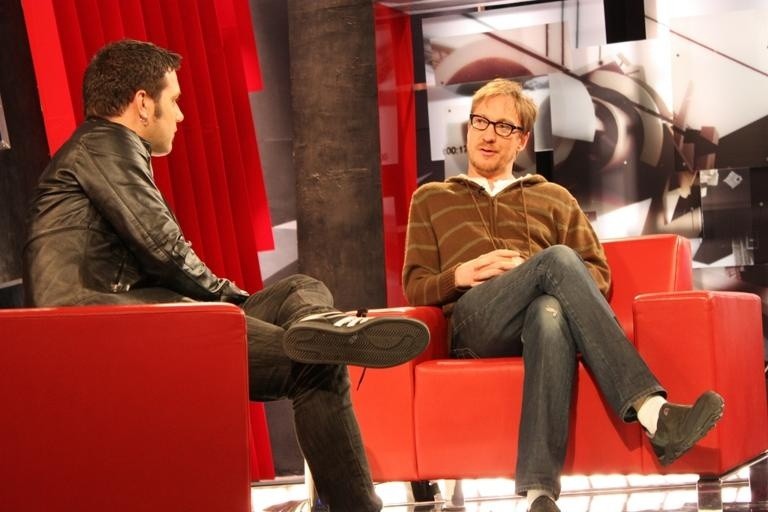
[347,235,768,512]
[0,302,275,512]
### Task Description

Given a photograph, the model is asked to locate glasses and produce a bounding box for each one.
[469,113,524,138]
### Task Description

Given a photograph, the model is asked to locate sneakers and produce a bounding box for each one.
[644,390,725,466]
[282,310,430,368]
[526,495,560,512]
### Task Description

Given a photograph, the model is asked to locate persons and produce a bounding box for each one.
[401,77,725,512]
[23,40,431,512]
